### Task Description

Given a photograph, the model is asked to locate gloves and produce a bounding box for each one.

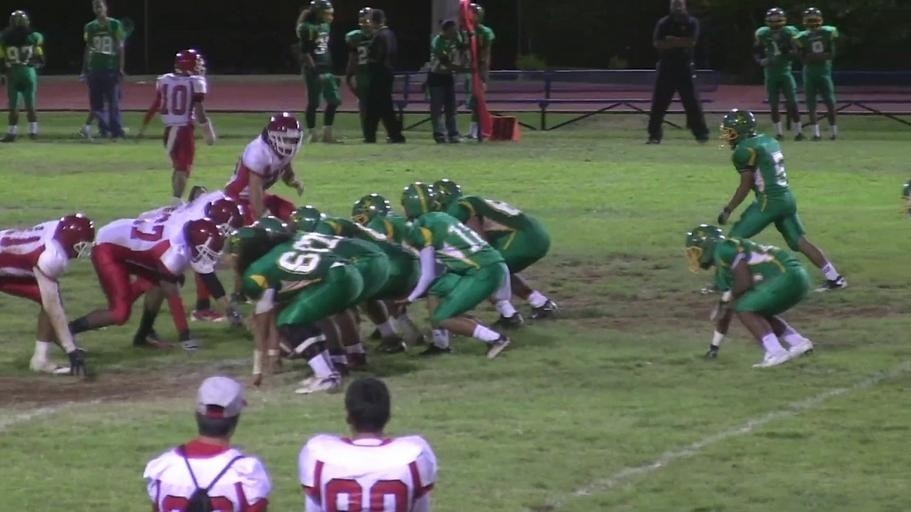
[196,116,216,146]
[718,206,731,225]
[705,343,718,360]
[68,347,93,377]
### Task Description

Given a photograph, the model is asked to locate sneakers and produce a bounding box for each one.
[293,326,453,394]
[811,136,821,141]
[30,357,71,374]
[133,334,175,349]
[449,133,462,143]
[814,275,848,293]
[493,312,525,330]
[789,338,813,358]
[76,124,95,143]
[0,134,15,142]
[485,333,510,360]
[191,308,228,322]
[752,348,792,368]
[775,134,784,141]
[527,298,558,320]
[699,285,731,295]
[794,134,808,141]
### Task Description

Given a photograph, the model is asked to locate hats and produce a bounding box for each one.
[194,376,248,420]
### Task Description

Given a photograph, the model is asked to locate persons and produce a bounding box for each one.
[68,215,245,352]
[142,187,244,324]
[295,1,345,145]
[224,196,423,393]
[0,10,48,143]
[401,184,524,359]
[344,7,376,134]
[79,0,130,139]
[143,376,272,510]
[429,17,469,143]
[79,16,137,141]
[791,8,839,140]
[898,180,911,228]
[361,8,407,144]
[138,48,218,201]
[431,179,559,330]
[752,7,808,142]
[0,214,100,381]
[699,108,849,297]
[297,377,440,511]
[644,1,711,146]
[224,110,308,230]
[683,226,817,370]
[462,3,496,140]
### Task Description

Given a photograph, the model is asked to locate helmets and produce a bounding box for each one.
[802,7,823,29]
[358,7,374,28]
[266,111,304,159]
[52,214,95,260]
[184,179,462,275]
[174,48,206,76]
[763,7,787,30]
[8,10,30,30]
[718,108,757,150]
[683,224,726,275]
[468,3,484,24]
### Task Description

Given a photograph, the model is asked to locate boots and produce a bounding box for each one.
[322,125,344,143]
[306,127,317,144]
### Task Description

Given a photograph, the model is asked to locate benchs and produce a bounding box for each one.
[392,68,717,130]
[763,69,910,130]
